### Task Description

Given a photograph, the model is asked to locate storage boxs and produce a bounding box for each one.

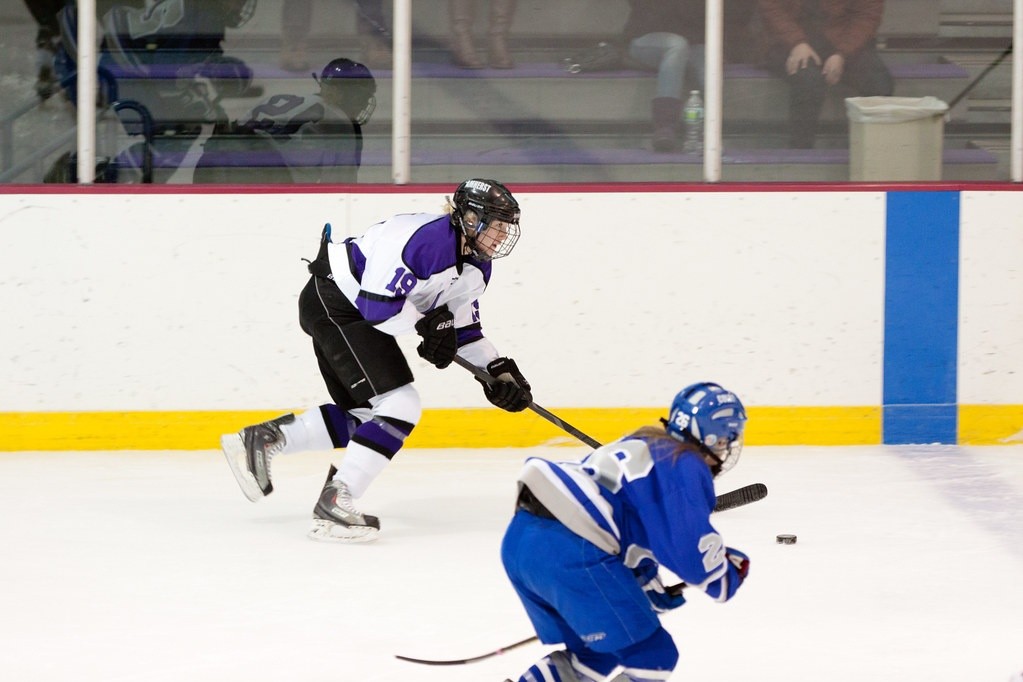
[845,93,949,182]
[682,90,704,157]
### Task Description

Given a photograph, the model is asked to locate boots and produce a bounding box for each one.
[448,0,484,69]
[486,0,518,69]
[651,96,682,149]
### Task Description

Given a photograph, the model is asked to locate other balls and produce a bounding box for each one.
[776,534,797,545]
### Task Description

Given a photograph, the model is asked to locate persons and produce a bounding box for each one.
[501,381,752,682]
[21,0,379,184]
[219,177,533,546]
[443,0,518,72]
[623,0,756,153]
[276,1,392,74]
[760,0,894,148]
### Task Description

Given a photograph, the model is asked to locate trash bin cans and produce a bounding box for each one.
[842,93,949,182]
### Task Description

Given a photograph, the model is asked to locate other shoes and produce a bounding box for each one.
[358,31,393,72]
[788,122,817,149]
[279,25,312,70]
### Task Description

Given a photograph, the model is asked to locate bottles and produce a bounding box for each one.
[680,90,703,156]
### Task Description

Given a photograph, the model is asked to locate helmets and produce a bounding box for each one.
[453,178,521,264]
[660,382,746,477]
[197,1,258,29]
[313,59,377,125]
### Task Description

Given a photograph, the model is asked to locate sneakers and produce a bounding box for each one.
[307,463,380,543]
[220,414,295,502]
[33,35,65,108]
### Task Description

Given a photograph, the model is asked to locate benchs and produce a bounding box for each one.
[43,49,972,126]
[86,128,1001,183]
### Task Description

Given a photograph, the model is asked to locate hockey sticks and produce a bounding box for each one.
[412,319,768,514]
[392,585,684,666]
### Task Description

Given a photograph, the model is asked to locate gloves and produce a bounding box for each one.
[414,303,458,369]
[475,357,533,412]
